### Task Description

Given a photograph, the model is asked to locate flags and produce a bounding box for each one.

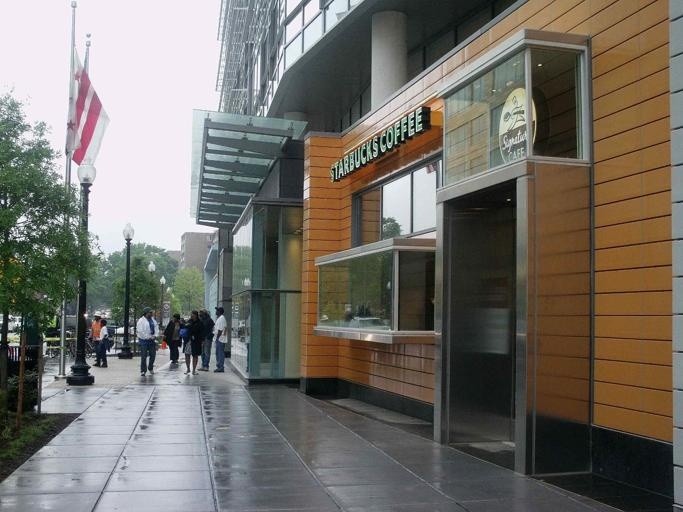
[64,42,109,168]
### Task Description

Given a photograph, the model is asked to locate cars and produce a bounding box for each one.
[0,312,117,332]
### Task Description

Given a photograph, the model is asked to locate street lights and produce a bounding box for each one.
[158,274,166,328]
[167,287,175,304]
[64,154,96,386]
[117,220,135,360]
[147,261,158,320]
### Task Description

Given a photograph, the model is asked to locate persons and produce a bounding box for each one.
[162,314,182,366]
[180,310,205,375]
[212,306,227,373]
[94,320,108,368]
[88,312,103,361]
[134,307,158,377]
[198,307,214,371]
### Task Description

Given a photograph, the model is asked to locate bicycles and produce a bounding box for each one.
[50,328,94,359]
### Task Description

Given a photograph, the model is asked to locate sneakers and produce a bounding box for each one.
[141,372,146,375]
[92,358,107,367]
[149,370,154,374]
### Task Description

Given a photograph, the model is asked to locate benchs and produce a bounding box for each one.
[8,345,49,368]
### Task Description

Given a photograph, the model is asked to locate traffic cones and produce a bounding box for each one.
[159,340,168,349]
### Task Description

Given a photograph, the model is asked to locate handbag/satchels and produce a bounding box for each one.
[179,328,189,337]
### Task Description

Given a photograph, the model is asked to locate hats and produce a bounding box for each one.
[93,311,101,317]
[143,307,153,315]
[215,307,224,313]
